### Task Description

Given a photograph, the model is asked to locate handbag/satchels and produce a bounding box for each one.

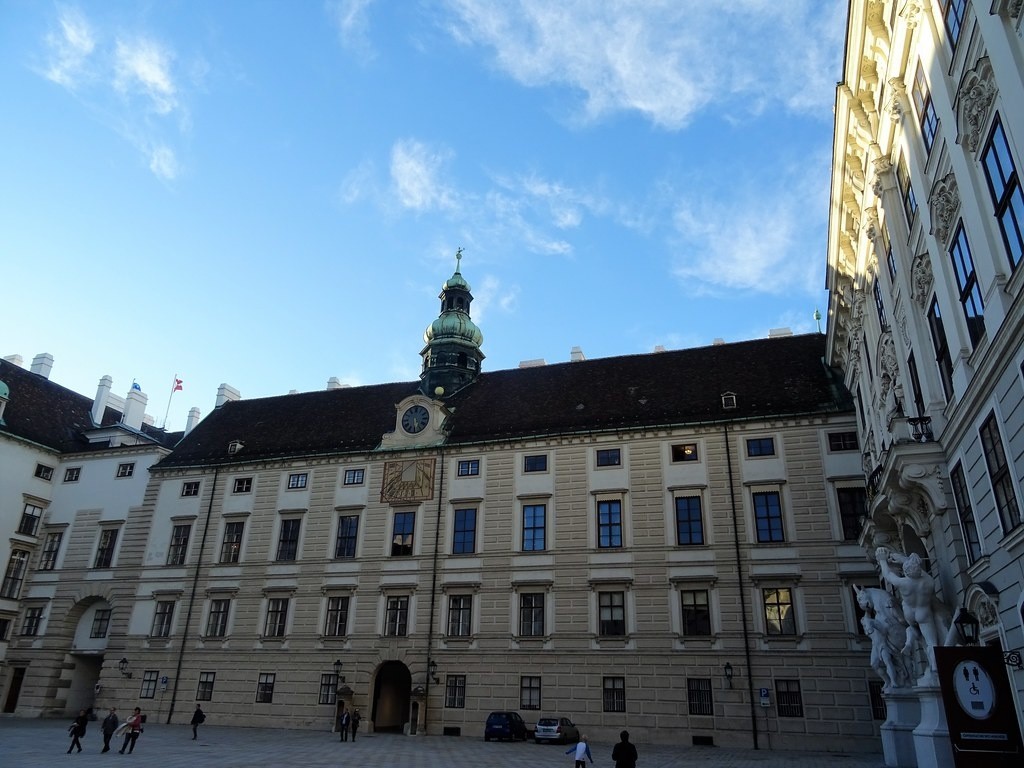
[141,728,143,733]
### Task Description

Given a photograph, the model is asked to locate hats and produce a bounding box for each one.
[355,707,360,711]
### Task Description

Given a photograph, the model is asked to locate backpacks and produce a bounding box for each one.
[199,713,207,723]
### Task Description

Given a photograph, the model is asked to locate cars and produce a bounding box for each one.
[485,712,528,741]
[535,717,580,745]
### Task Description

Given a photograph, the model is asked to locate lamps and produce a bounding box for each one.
[723,662,732,687]
[682,446,695,454]
[118,658,132,678]
[428,661,439,684]
[334,659,345,683]
[954,607,1023,669]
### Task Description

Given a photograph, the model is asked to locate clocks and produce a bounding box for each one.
[399,403,432,438]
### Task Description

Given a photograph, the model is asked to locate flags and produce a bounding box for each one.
[173,379,183,392]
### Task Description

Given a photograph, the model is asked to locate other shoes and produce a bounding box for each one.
[100,748,110,754]
[192,736,197,740]
[345,739,347,742]
[127,750,132,754]
[352,739,356,742]
[119,749,124,754]
[77,749,82,754]
[340,739,343,742]
[67,751,71,754]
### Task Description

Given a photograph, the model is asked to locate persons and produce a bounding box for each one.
[875,547,940,672]
[565,735,594,768]
[340,708,361,742]
[861,613,899,691]
[612,730,638,768]
[101,707,118,754]
[67,710,88,754]
[880,370,904,429]
[118,707,141,754]
[190,704,203,740]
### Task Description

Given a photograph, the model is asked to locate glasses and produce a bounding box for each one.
[110,710,114,711]
